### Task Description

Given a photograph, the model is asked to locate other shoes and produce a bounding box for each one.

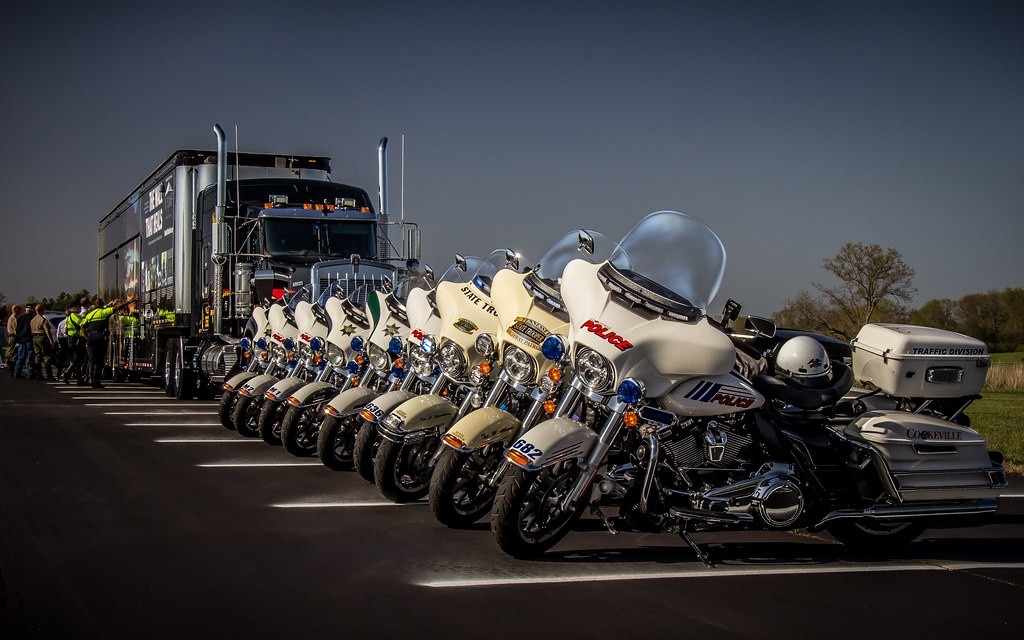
[8,369,105,389]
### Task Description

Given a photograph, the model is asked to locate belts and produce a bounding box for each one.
[33,333,46,336]
[9,334,17,337]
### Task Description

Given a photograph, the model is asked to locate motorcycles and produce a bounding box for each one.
[352,255,497,484]
[260,278,352,446]
[374,248,544,503]
[317,275,421,471]
[489,209,1007,569]
[429,228,634,527]
[280,284,379,457]
[218,298,268,430]
[233,282,323,437]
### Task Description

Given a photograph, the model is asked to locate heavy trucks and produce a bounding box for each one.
[92,122,425,401]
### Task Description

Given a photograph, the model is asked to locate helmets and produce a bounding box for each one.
[775,335,833,387]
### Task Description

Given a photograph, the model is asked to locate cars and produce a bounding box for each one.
[43,313,67,348]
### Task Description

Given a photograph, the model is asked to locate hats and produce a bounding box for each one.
[70,301,84,308]
[25,304,35,309]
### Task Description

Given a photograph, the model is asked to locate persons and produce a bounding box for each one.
[0,296,146,390]
